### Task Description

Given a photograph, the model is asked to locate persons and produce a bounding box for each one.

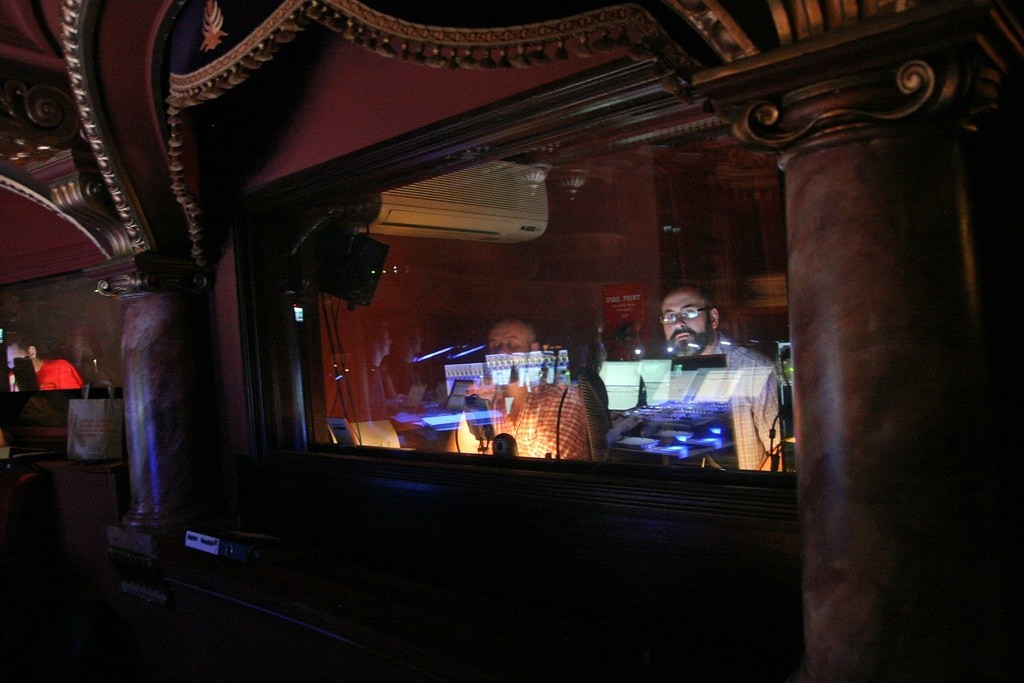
[4,328,86,392]
[651,279,782,472]
[447,316,591,461]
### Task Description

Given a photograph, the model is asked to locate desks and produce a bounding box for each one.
[37,458,133,630]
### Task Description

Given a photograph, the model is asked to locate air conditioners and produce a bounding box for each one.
[359,160,549,245]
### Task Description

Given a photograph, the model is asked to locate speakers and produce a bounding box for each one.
[314,224,389,306]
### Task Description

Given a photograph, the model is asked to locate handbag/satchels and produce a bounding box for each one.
[68,381,124,460]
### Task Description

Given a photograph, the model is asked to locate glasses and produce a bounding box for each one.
[660,306,713,324]
